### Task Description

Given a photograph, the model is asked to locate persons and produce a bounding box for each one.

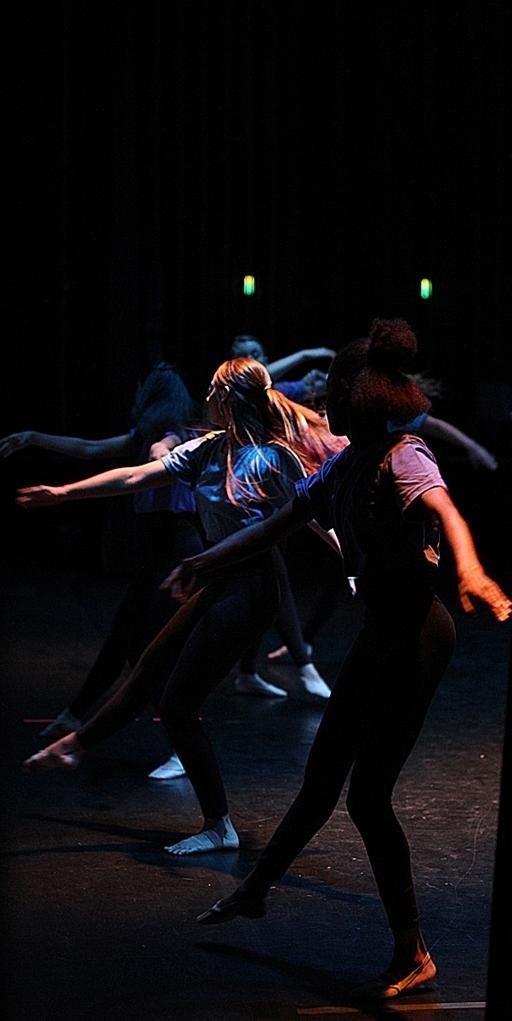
[231,334,345,702]
[0,369,194,781]
[159,319,512,1005]
[265,377,500,667]
[17,358,307,855]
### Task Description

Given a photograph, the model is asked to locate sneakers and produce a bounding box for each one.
[148,757,186,779]
[295,674,331,698]
[235,678,287,697]
[195,898,267,925]
[365,952,438,1001]
